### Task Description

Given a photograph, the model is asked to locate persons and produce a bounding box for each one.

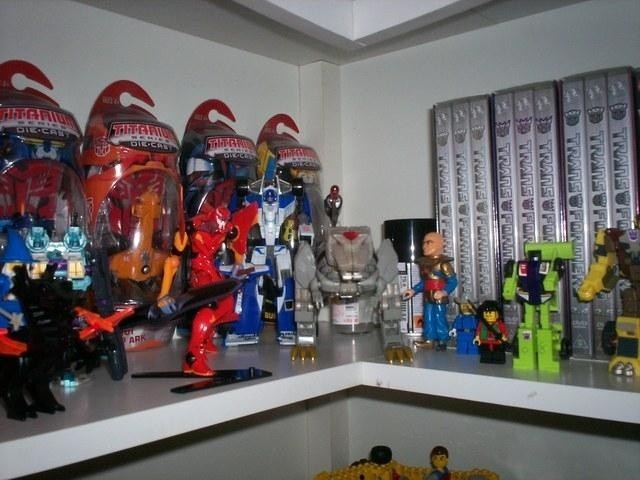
[397,231,458,352]
[1,56,416,425]
